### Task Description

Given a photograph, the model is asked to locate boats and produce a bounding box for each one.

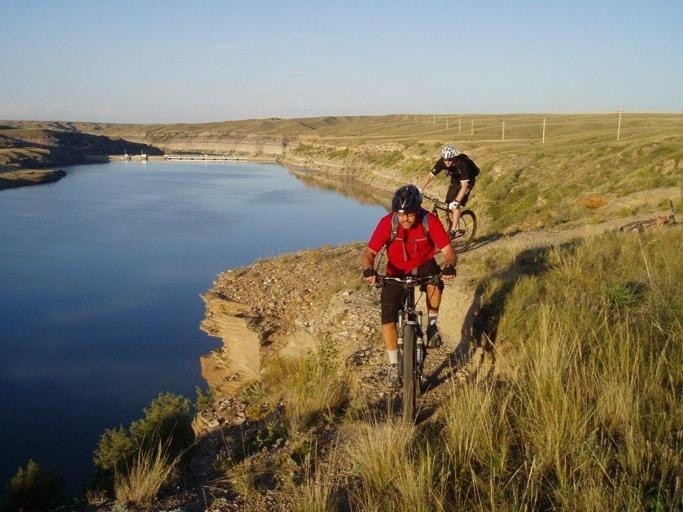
[106,145,148,162]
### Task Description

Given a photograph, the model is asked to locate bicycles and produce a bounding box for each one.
[419,193,479,256]
[367,272,445,425]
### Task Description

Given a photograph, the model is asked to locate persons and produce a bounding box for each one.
[359,184,457,388]
[419,144,479,241]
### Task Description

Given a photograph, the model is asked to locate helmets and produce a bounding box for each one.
[392,183,422,212]
[440,146,457,159]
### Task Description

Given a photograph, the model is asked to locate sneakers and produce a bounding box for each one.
[447,230,455,239]
[426,325,442,348]
[386,362,403,388]
